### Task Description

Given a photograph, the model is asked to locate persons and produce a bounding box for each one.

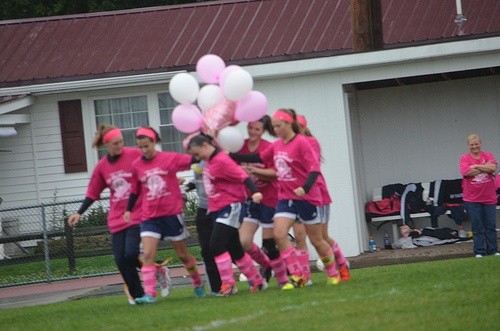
[67,108,351,306]
[122,127,207,304]
[459,134,500,258]
[186,133,268,297]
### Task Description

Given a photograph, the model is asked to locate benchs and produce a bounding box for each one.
[371,174,500,248]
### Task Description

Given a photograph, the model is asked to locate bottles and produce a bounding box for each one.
[369,236,377,251]
[384,233,392,249]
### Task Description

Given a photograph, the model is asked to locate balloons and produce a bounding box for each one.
[169,53,268,154]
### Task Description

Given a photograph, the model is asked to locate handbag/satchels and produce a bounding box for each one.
[365,194,411,218]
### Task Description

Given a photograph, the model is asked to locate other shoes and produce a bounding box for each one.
[340,261,350,280]
[494,252,500,255]
[135,294,157,304]
[326,274,340,285]
[476,254,482,258]
[157,265,171,299]
[210,267,312,296]
[193,284,205,297]
